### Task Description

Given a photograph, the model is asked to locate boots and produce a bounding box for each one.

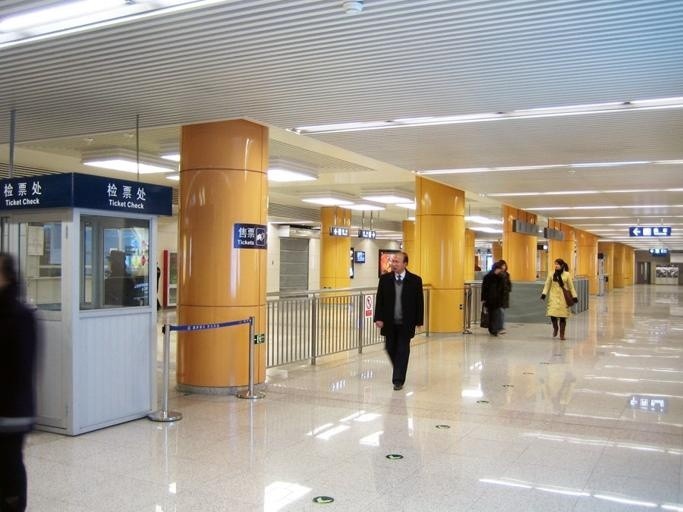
[552,321,559,337]
[559,322,566,340]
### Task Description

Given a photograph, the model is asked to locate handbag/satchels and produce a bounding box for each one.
[480,312,489,328]
[564,290,574,306]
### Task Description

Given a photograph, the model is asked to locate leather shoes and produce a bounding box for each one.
[394,383,403,390]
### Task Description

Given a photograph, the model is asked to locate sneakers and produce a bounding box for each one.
[490,328,507,337]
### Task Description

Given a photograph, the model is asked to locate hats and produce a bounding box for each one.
[106,250,126,261]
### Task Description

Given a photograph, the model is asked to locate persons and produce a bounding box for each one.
[373,252,425,390]
[479,259,512,336]
[105,250,135,306]
[541,258,579,340]
[1,253,37,512]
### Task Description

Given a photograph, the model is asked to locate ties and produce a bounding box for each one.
[397,275,401,282]
